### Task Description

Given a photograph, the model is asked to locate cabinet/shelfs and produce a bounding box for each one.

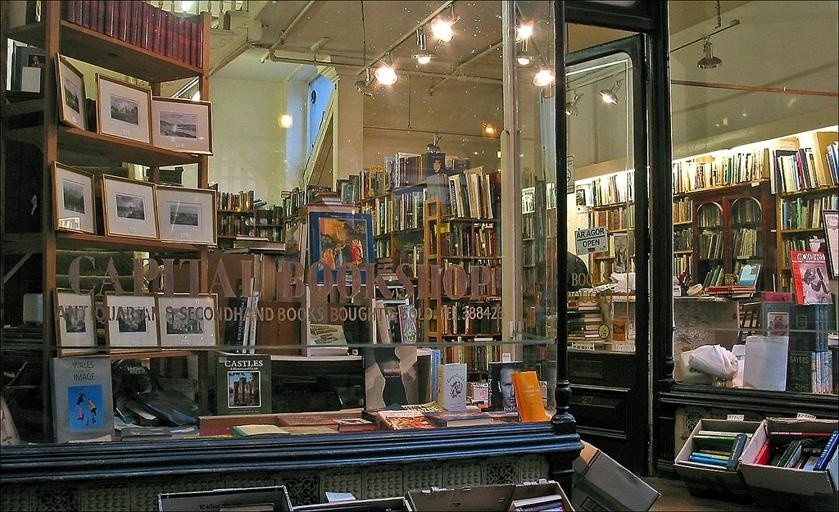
[778,128,838,304]
[215,182,340,356]
[363,156,549,371]
[562,154,692,383]
[0,0,220,444]
[695,137,788,382]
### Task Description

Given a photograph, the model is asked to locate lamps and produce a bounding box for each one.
[428,17,456,46]
[563,92,582,116]
[531,65,556,87]
[697,39,723,71]
[516,18,537,42]
[410,47,436,66]
[595,81,621,107]
[516,43,531,65]
[376,60,397,86]
[357,64,377,98]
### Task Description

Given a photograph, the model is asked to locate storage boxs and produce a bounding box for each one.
[294,496,411,512]
[570,436,663,512]
[406,481,572,512]
[738,419,839,512]
[673,414,762,504]
[158,485,294,512]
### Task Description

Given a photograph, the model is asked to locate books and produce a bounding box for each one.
[754,431,839,470]
[674,228,692,251]
[673,197,691,223]
[402,400,446,415]
[703,266,725,285]
[567,300,605,341]
[817,350,833,395]
[820,303,836,351]
[733,228,756,259]
[698,230,724,259]
[357,152,470,197]
[430,303,503,335]
[789,305,820,351]
[587,205,634,229]
[673,255,692,282]
[234,235,285,254]
[377,409,437,432]
[442,346,502,370]
[215,353,272,415]
[788,351,816,394]
[438,364,466,413]
[704,285,756,298]
[309,212,374,288]
[487,361,526,412]
[433,222,497,256]
[423,409,493,426]
[442,259,504,295]
[426,166,503,218]
[677,430,753,470]
[219,502,274,512]
[281,426,339,437]
[781,273,795,303]
[506,494,566,512]
[673,148,770,194]
[60,1,202,70]
[417,349,442,400]
[760,291,791,336]
[511,371,547,422]
[233,424,288,439]
[361,189,430,235]
[590,169,635,206]
[212,183,253,211]
[332,416,381,431]
[225,292,259,354]
[733,199,761,222]
[217,214,255,236]
[361,403,406,421]
[48,356,115,443]
[256,206,285,241]
[307,324,348,355]
[281,185,343,213]
[275,414,339,427]
[769,131,839,194]
[788,249,832,304]
[697,204,724,227]
[779,194,838,230]
[738,304,761,341]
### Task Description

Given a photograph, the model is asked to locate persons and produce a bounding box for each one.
[785,237,825,266]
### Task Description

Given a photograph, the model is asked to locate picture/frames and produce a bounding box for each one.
[154,186,218,246]
[100,173,159,240]
[152,94,212,157]
[159,292,219,348]
[53,53,90,130]
[94,72,153,144]
[52,287,97,357]
[104,288,162,356]
[51,160,96,236]
